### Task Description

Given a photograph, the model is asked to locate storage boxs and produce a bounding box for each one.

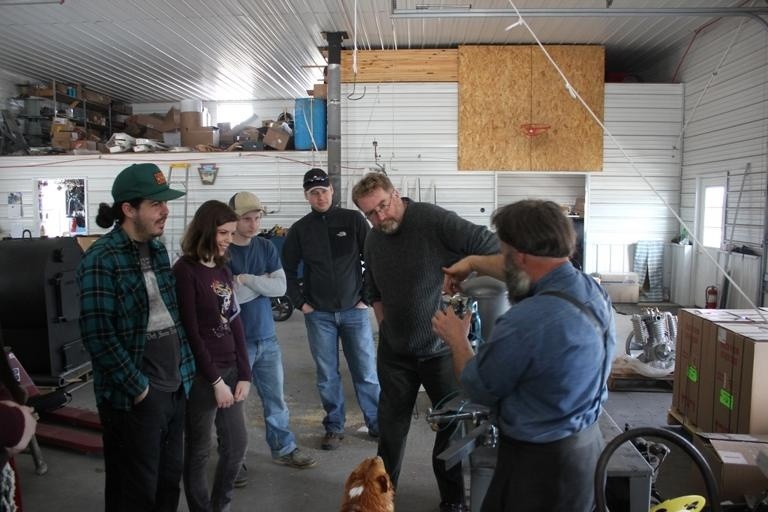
[595,272,640,303]
[50,111,290,151]
[671,309,768,507]
[306,84,328,99]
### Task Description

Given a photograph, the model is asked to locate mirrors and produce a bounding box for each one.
[34,176,89,238]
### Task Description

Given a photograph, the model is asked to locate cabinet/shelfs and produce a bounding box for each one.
[16,78,133,143]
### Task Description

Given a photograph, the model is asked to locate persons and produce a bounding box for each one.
[77,163,198,511]
[224,191,317,488]
[352,174,502,510]
[172,199,251,512]
[431,199,616,511]
[282,168,379,451]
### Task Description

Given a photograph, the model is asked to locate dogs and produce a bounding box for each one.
[338,456,398,512]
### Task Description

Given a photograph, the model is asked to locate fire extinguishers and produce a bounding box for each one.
[705,286,717,308]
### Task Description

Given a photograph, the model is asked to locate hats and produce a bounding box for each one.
[229,191,267,216]
[112,163,186,202]
[303,168,330,194]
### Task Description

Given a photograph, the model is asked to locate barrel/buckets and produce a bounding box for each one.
[23,97,41,116]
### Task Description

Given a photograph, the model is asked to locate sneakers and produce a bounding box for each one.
[322,430,345,450]
[272,447,318,469]
[232,463,249,487]
[368,429,382,440]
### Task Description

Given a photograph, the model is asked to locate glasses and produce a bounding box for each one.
[364,194,398,219]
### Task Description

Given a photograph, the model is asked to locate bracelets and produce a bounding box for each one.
[209,375,222,386]
[237,273,242,285]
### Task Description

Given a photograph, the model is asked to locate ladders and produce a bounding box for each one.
[159,161,191,267]
